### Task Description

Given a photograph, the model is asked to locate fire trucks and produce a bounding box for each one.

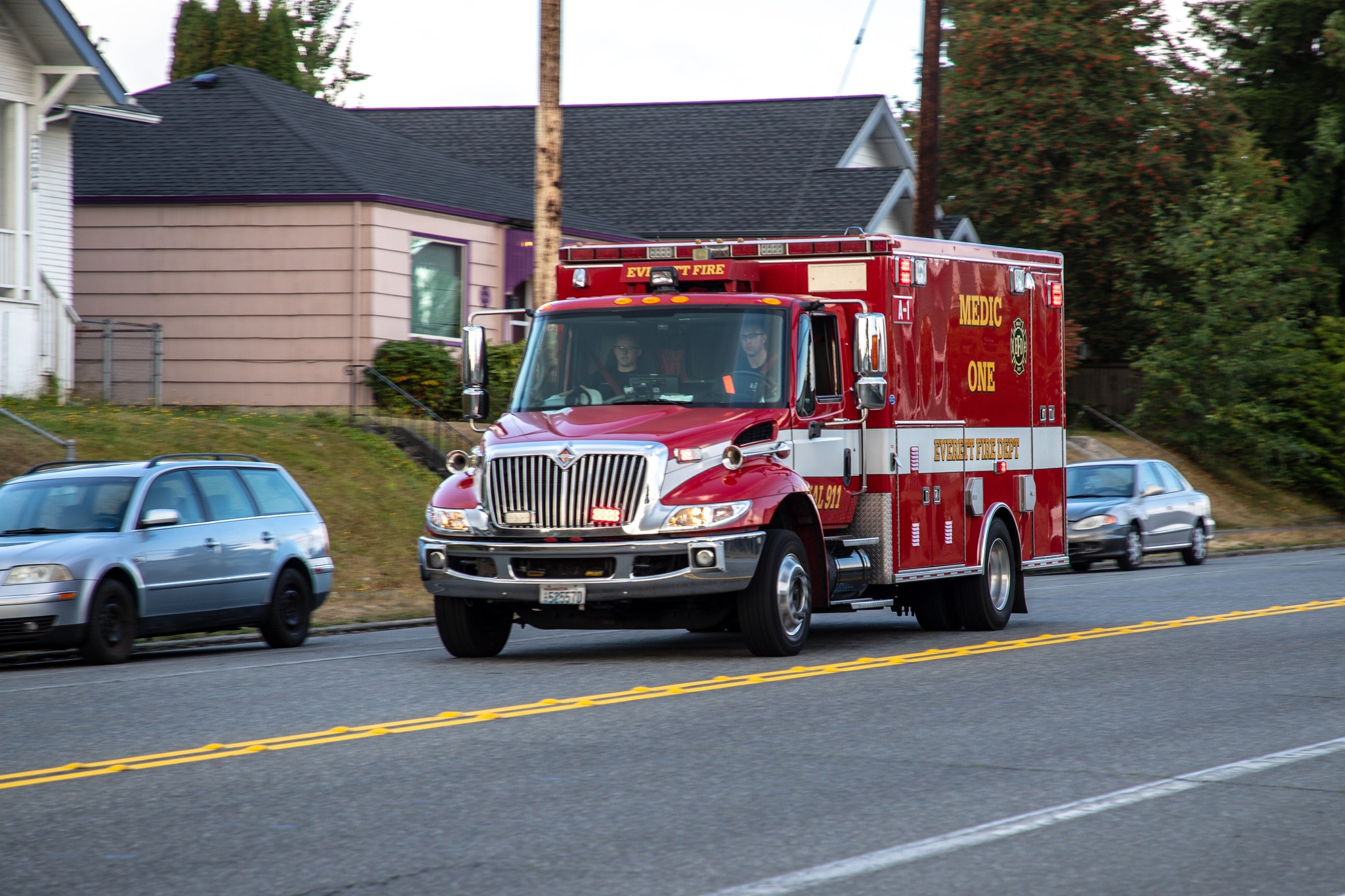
[415,231,1069,660]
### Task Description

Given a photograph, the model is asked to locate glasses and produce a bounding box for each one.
[615,346,641,353]
[740,333,765,340]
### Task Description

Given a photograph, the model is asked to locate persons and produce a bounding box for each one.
[545,332,650,401]
[711,319,779,400]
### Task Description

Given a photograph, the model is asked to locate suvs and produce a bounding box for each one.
[0,452,336,667]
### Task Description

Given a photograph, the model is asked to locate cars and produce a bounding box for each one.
[1066,459,1218,577]
